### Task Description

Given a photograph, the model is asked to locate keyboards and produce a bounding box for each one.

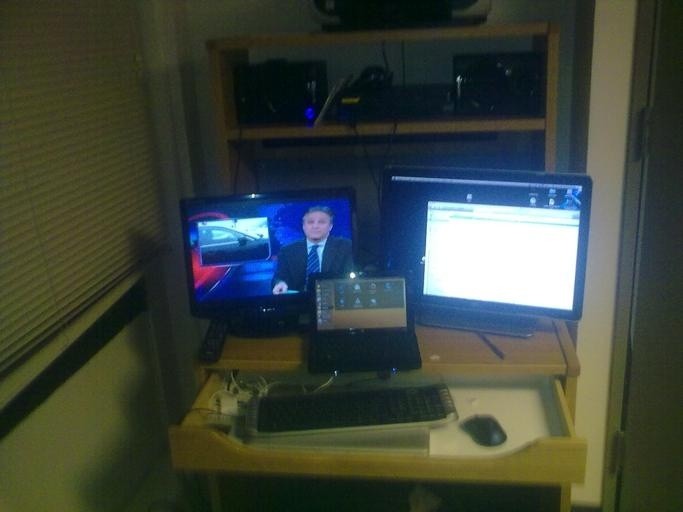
[245,385,456,433]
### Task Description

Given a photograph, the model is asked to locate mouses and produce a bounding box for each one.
[467,417,509,445]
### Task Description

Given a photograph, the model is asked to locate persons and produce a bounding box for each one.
[271,206,353,295]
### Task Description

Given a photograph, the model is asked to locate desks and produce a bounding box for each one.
[172,314,588,510]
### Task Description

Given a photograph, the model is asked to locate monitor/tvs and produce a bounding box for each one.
[382,168,594,334]
[175,186,359,337]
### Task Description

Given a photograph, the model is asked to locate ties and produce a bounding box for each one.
[304,245,320,286]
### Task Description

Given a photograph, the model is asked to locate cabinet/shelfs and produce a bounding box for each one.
[206,20,559,198]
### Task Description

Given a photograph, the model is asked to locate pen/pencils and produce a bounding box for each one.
[477,332,507,360]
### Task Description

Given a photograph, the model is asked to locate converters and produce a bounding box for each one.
[214,391,238,416]
